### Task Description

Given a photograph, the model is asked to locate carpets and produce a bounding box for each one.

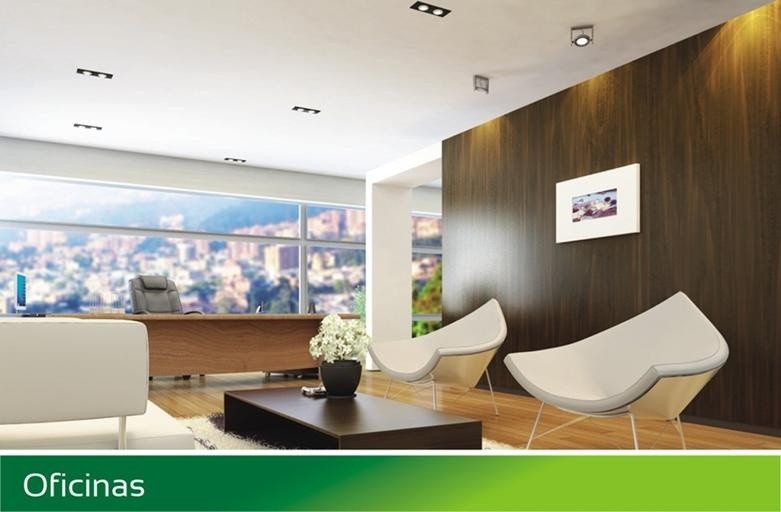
[174,413,522,448]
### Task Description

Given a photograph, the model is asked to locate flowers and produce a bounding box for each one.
[307,312,373,363]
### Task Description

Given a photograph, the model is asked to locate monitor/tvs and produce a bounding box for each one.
[14,272,28,313]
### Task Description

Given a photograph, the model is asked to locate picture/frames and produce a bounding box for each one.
[553,162,641,243]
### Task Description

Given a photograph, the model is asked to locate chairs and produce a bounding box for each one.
[365,296,509,418]
[128,275,205,382]
[0,317,194,449]
[502,289,731,451]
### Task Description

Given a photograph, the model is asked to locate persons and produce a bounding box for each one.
[573,195,617,222]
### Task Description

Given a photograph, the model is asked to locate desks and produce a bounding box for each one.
[49,313,360,382]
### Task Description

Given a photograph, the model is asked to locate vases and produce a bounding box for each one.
[320,360,363,400]
